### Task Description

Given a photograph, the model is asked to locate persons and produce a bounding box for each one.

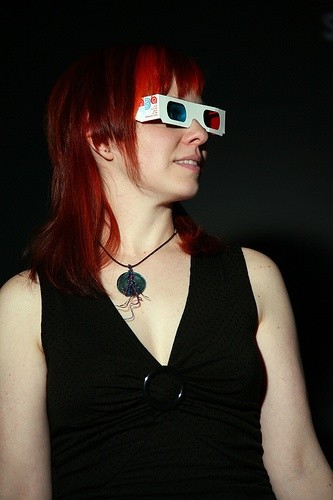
[0,30,333,500]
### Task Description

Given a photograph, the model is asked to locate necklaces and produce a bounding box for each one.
[93,227,180,297]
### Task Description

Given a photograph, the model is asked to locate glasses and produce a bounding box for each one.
[135,94,227,137]
